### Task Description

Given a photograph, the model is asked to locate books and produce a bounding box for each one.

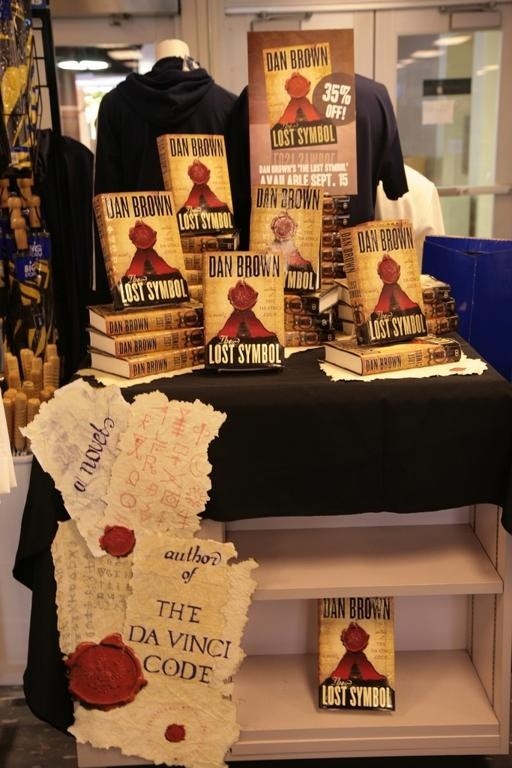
[154,134,237,235]
[284,306,333,331]
[91,191,190,312]
[252,184,322,292]
[337,300,455,321]
[324,334,462,377]
[323,263,345,278]
[248,30,357,196]
[85,327,203,356]
[322,216,348,231]
[183,254,202,270]
[179,232,238,254]
[284,332,337,346]
[187,286,203,302]
[285,281,342,311]
[338,221,428,346]
[187,268,203,284]
[334,275,450,305]
[89,304,197,335]
[202,251,284,371]
[323,197,349,214]
[343,316,457,336]
[317,596,398,714]
[321,247,344,264]
[91,350,203,378]
[322,232,341,248]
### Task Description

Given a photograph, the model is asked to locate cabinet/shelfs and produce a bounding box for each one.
[15,324,511,763]
